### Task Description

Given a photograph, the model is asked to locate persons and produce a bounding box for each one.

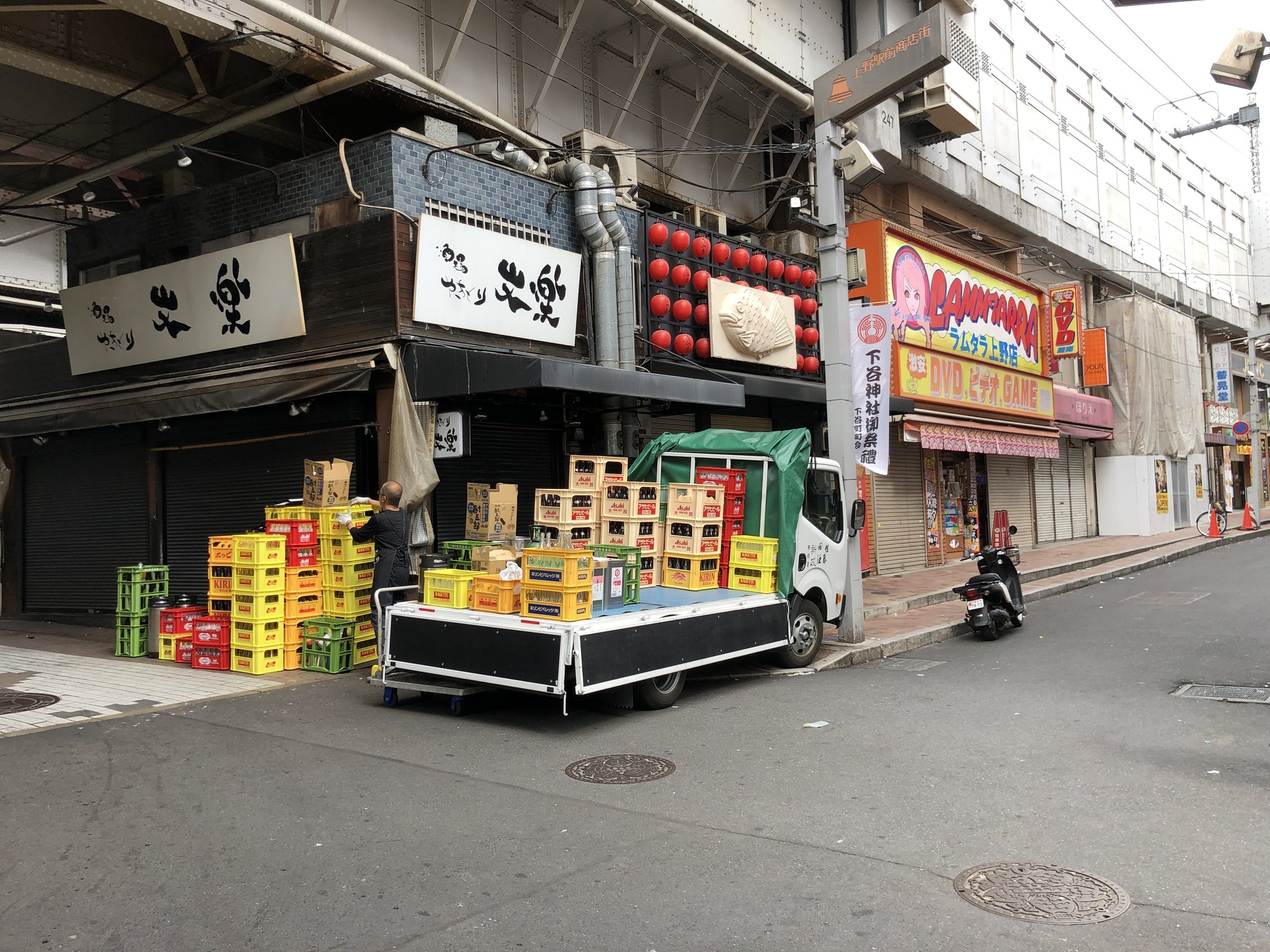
[337,480,411,675]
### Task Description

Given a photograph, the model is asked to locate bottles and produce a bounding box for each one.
[272,512,378,653]
[339,628,352,668]
[526,533,588,604]
[212,566,232,611]
[236,541,278,658]
[667,471,777,578]
[165,612,221,656]
[431,577,472,591]
[451,550,471,570]
[540,461,655,570]
[127,571,165,609]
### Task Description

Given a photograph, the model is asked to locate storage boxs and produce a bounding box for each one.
[116,565,169,658]
[424,454,779,622]
[159,457,378,676]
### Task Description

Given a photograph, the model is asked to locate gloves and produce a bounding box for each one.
[350,497,370,506]
[336,508,352,526]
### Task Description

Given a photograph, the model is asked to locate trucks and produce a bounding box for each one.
[380,427,866,716]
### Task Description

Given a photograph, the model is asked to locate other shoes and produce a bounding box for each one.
[366,666,395,681]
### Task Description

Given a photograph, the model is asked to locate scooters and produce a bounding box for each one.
[950,526,1028,641]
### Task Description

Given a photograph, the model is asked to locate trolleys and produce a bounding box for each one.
[371,585,494,716]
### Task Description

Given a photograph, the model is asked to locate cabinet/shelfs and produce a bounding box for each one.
[943,462,967,498]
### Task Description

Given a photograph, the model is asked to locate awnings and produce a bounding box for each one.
[401,337,746,415]
[1054,389,1114,443]
[0,342,398,438]
[889,414,1060,460]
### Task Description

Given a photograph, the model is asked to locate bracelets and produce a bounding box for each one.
[346,522,352,527]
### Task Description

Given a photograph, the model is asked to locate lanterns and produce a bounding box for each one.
[649,222,819,373]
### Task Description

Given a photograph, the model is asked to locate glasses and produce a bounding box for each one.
[377,490,387,498]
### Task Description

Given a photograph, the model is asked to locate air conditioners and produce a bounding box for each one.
[562,129,638,209]
[663,205,751,243]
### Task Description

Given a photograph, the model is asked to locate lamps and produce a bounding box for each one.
[1014,263,1066,275]
[77,181,177,219]
[569,411,581,427]
[44,297,61,312]
[547,184,640,215]
[172,142,282,196]
[1231,339,1249,346]
[833,140,885,190]
[926,227,982,241]
[475,406,486,421]
[1088,438,1096,448]
[422,137,508,180]
[290,398,314,416]
[1245,342,1270,355]
[1236,339,1261,351]
[159,419,170,433]
[974,247,1028,259]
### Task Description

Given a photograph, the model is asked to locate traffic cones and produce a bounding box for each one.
[1204,509,1225,539]
[1236,503,1255,531]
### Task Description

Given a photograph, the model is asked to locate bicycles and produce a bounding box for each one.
[1195,490,1229,537]
